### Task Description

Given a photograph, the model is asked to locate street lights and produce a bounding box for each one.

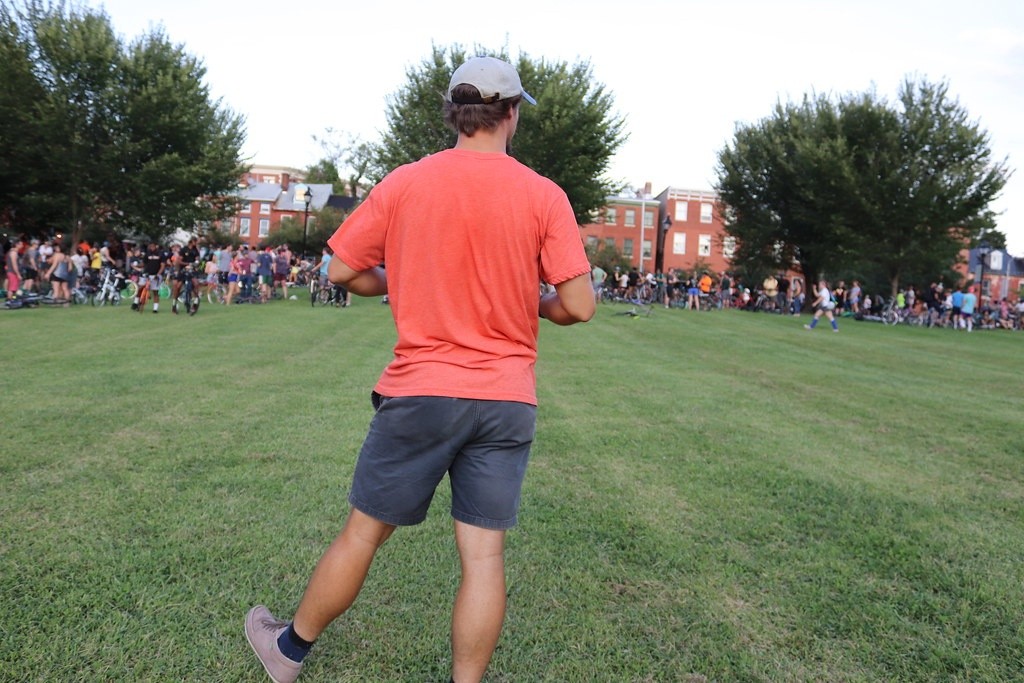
[979,241,990,311]
[302,187,313,252]
[659,215,672,273]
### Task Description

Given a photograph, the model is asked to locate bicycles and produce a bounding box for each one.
[6,267,227,313]
[601,283,769,312]
[882,296,899,326]
[311,284,347,308]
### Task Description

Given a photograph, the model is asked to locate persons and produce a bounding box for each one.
[0,233,390,316]
[537,264,1024,333]
[245,55,596,683]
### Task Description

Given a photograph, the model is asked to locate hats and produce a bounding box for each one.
[266,247,270,252]
[243,250,249,255]
[448,56,537,106]
[744,288,750,293]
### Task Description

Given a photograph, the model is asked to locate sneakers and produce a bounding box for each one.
[244,605,303,682]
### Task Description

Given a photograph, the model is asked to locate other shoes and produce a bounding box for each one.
[833,329,838,332]
[804,324,811,329]
[131,303,137,310]
[153,310,157,313]
[793,313,801,317]
[192,303,197,312]
[172,305,176,312]
